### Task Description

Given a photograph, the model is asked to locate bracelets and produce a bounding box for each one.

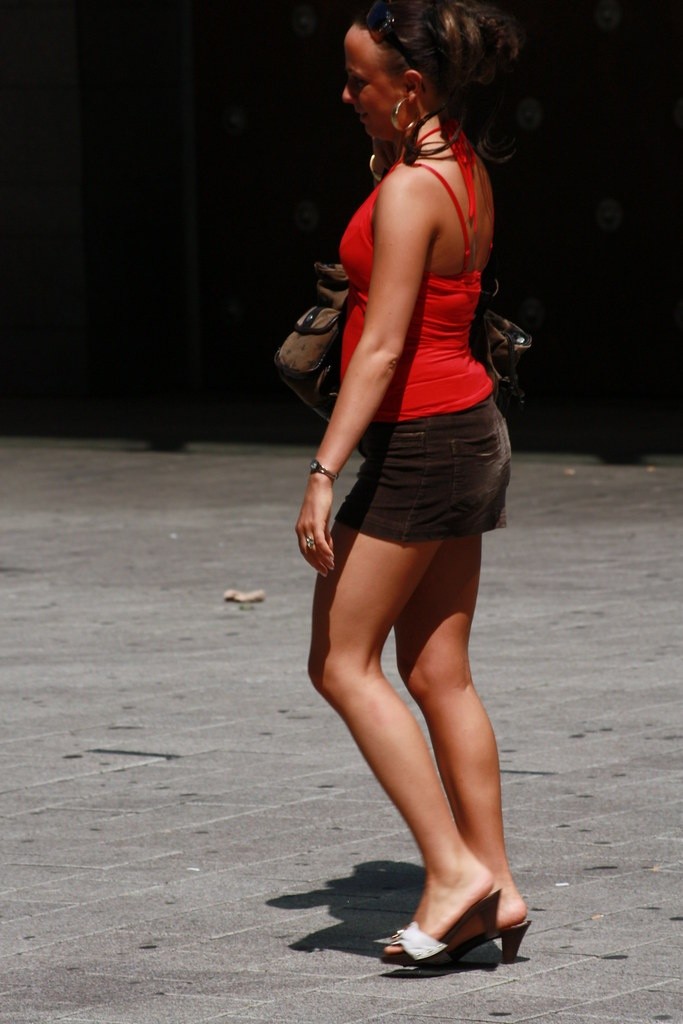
[369,154,381,182]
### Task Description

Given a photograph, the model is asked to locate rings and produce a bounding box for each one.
[307,537,314,549]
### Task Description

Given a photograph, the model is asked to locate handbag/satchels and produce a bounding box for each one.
[277,263,533,422]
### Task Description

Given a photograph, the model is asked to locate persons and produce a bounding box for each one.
[294,0,531,967]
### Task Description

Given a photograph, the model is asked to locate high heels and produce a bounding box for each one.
[375,890,505,964]
[410,920,533,967]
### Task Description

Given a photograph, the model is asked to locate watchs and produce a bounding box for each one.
[310,459,335,479]
